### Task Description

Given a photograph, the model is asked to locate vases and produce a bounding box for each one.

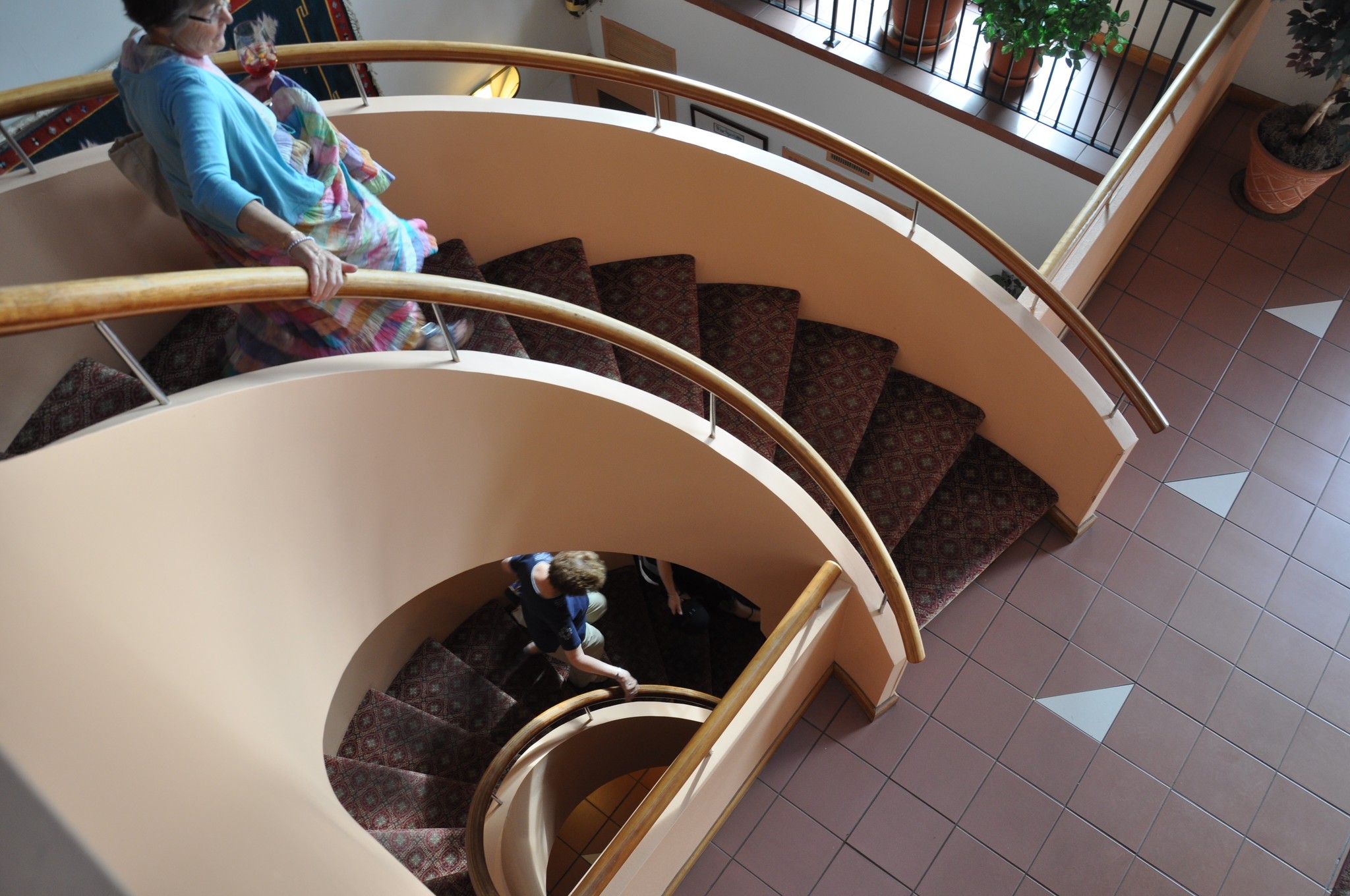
[880,0,966,54]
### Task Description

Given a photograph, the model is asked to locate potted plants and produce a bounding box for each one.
[973,0,1130,86]
[1247,0,1350,222]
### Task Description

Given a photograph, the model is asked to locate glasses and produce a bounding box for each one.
[181,0,231,26]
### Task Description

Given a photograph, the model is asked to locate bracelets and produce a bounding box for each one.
[286,236,315,261]
[667,592,679,598]
[614,667,621,680]
[744,606,754,620]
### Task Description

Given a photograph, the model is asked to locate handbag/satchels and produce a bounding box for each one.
[107,131,186,223]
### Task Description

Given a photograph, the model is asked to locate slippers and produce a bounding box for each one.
[420,316,476,351]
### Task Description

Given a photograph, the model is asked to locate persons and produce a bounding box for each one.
[499,550,640,704]
[693,571,762,624]
[632,552,710,633]
[110,1,476,384]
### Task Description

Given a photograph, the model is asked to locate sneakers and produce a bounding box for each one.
[523,640,537,658]
